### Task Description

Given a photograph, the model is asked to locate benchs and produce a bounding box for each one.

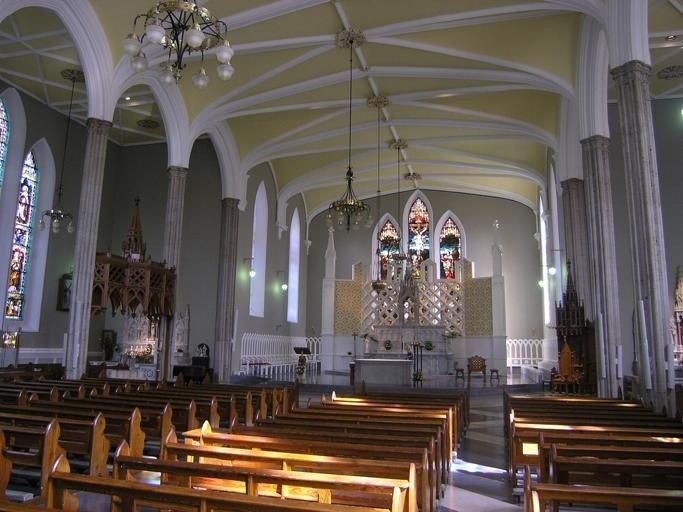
[0,362,471,512]
[501,387,683,512]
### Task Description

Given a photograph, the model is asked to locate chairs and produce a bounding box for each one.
[465,355,487,379]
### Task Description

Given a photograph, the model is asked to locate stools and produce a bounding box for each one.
[489,368,499,379]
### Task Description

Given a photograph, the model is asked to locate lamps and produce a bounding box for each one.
[36,68,86,234]
[122,0,235,90]
[323,30,374,233]
